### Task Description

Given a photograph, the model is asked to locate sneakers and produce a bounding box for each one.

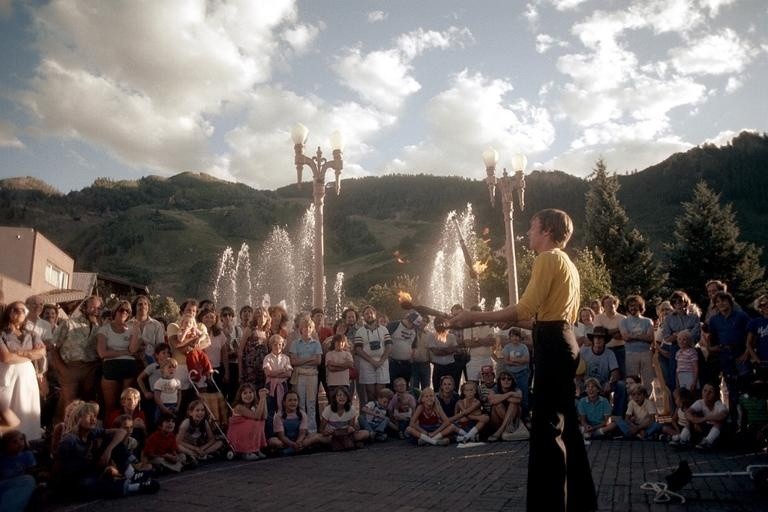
[407,432,480,446]
[487,433,501,441]
[669,437,691,447]
[434,316,446,328]
[129,469,160,494]
[408,311,423,331]
[695,437,713,449]
[183,455,193,464]
[242,450,266,460]
[623,433,641,440]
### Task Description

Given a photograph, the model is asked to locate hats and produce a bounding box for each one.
[587,327,613,344]
[758,295,768,308]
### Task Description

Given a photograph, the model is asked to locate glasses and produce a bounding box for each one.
[12,307,27,313]
[223,312,232,317]
[118,307,131,314]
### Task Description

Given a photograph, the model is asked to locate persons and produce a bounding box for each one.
[3,276,767,510]
[444,207,598,512]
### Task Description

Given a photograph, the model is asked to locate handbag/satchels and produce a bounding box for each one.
[453,352,471,364]
[37,373,50,397]
[332,428,355,451]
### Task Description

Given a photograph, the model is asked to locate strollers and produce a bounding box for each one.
[187,368,267,463]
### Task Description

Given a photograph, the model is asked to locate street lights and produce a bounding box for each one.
[290,118,345,317]
[479,145,532,306]
